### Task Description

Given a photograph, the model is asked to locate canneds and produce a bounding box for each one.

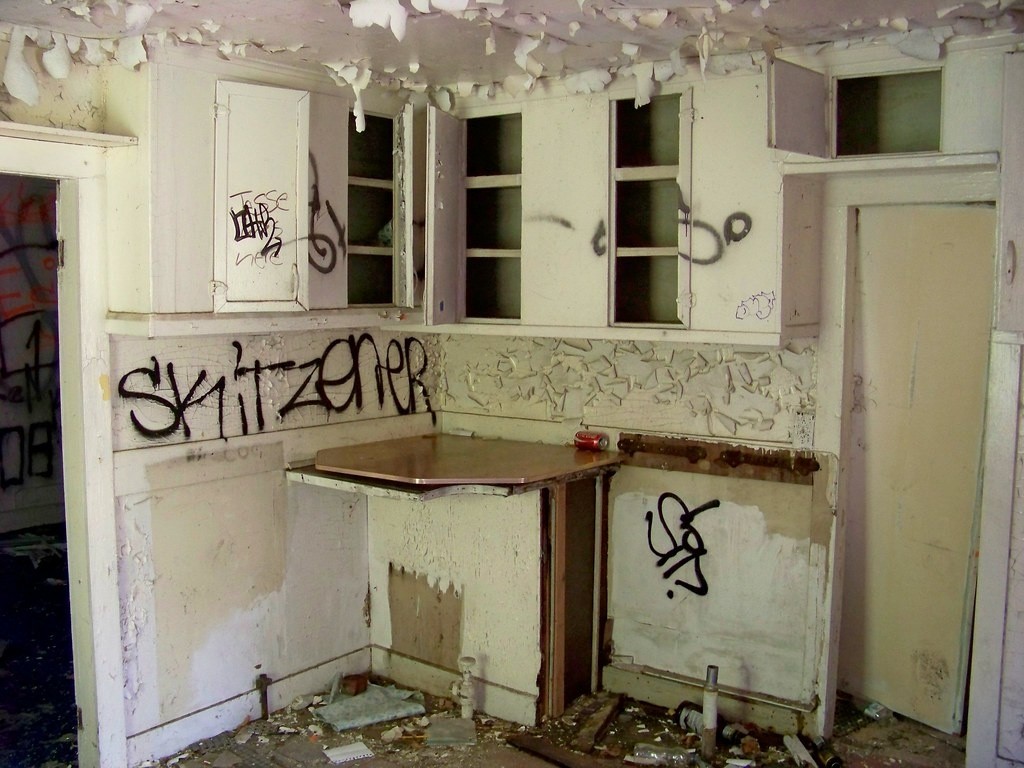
[574,431,609,451]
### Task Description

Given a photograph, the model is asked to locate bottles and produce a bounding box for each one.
[633,742,701,768]
[675,699,745,745]
[853,697,898,727]
[811,735,844,768]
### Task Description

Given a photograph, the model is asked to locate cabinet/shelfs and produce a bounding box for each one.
[607,74,820,345]
[380,90,611,339]
[98,62,426,340]
